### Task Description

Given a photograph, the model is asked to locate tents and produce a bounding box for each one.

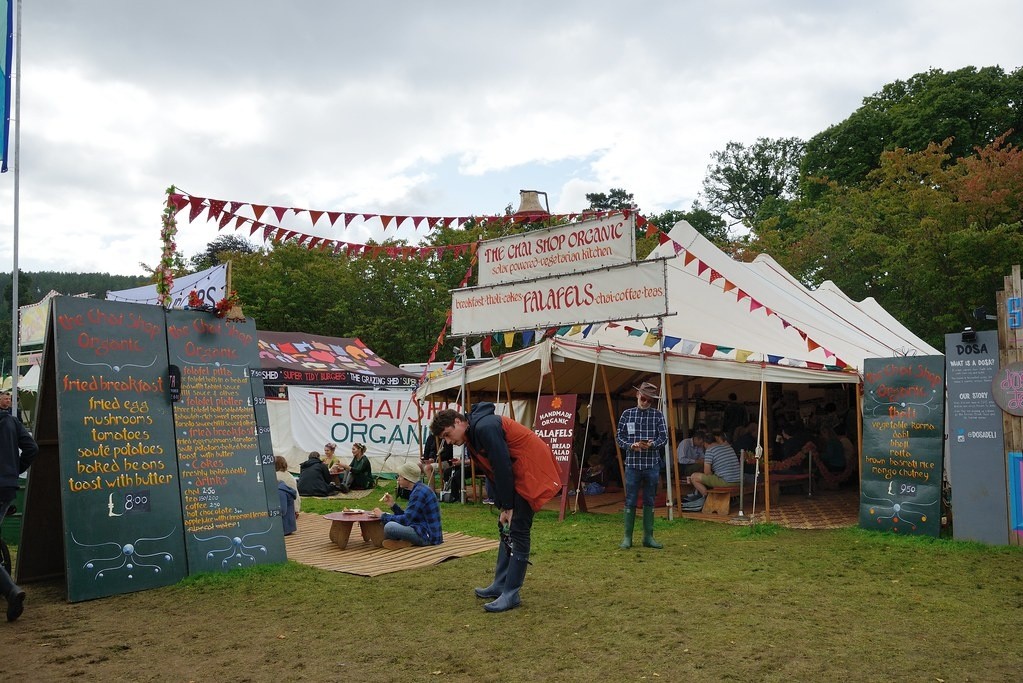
[255,221,950,525]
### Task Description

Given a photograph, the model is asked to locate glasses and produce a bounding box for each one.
[638,393,654,404]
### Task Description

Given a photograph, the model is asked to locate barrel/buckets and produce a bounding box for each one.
[0,477,27,546]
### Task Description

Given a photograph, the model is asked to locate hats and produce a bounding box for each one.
[398,462,421,484]
[633,382,662,399]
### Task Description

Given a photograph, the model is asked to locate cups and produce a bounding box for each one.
[687,477,691,484]
[776,435,782,442]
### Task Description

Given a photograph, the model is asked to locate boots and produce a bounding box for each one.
[484,549,533,612]
[642,505,663,549]
[620,505,635,549]
[475,543,512,598]
[0,564,25,622]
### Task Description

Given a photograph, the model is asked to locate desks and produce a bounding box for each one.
[322,512,387,552]
[329,471,347,486]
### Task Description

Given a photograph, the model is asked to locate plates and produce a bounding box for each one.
[367,512,390,517]
[342,509,365,513]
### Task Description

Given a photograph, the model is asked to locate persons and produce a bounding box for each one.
[273,382,854,611]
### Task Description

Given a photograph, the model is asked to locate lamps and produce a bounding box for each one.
[961,326,977,343]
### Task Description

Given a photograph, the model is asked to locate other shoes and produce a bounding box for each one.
[382,540,413,551]
[340,482,350,493]
[444,497,461,503]
[482,499,495,505]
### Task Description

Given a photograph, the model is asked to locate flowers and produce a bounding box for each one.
[747,442,830,483]
[136,186,246,325]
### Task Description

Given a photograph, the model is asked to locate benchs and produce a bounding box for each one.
[701,486,763,513]
[768,474,816,509]
